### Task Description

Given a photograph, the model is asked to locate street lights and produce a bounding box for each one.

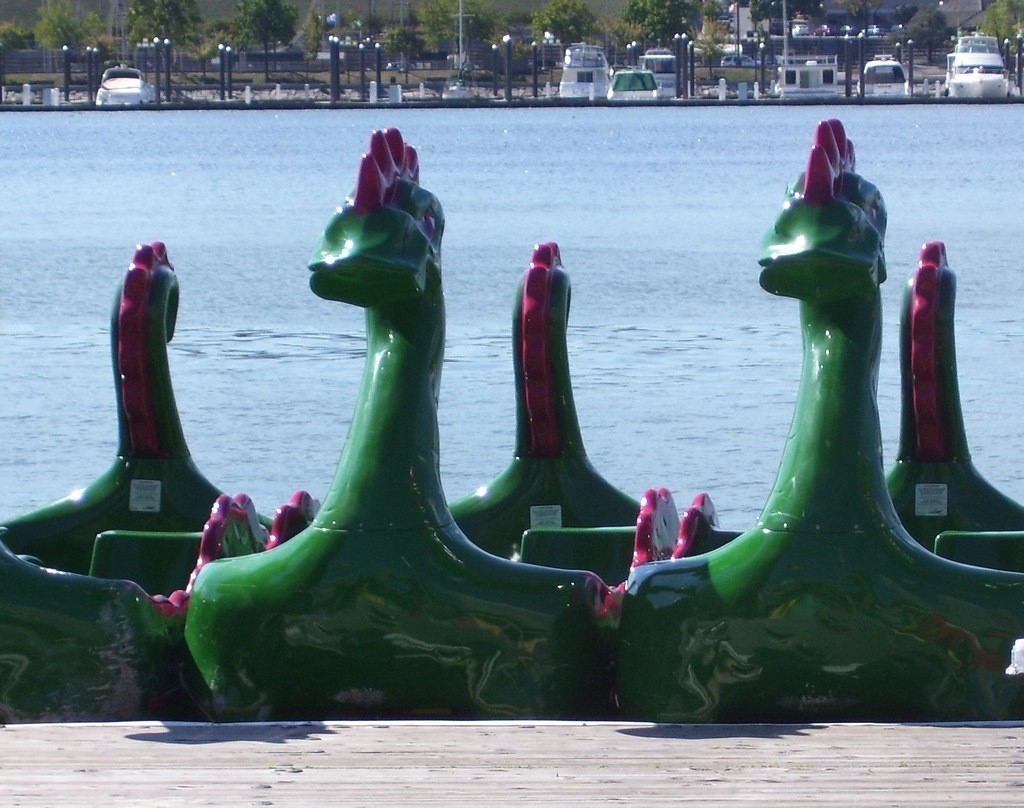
[63,46,72,101]
[329,35,341,104]
[153,38,171,106]
[844,35,1024,97]
[86,46,100,104]
[218,44,233,101]
[359,31,766,102]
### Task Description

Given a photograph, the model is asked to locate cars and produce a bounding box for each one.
[812,25,836,37]
[721,55,754,68]
[859,25,886,36]
[791,24,810,36]
[838,24,858,36]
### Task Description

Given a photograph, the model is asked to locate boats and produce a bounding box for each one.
[855,54,911,100]
[607,66,657,103]
[96,64,156,107]
[443,79,481,104]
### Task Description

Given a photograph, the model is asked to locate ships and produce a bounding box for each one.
[944,36,1010,99]
[559,41,611,98]
